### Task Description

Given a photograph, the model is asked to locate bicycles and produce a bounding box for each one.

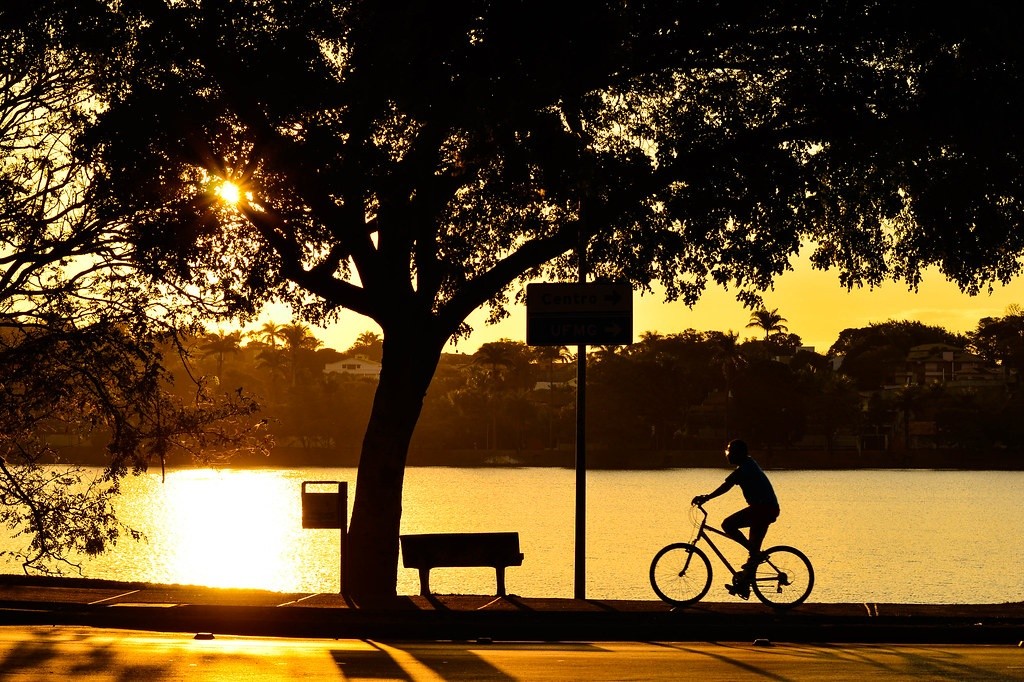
[650,493,814,611]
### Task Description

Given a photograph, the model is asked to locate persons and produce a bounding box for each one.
[689,439,780,598]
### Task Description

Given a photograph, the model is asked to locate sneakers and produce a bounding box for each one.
[725,584,750,598]
[741,552,770,568]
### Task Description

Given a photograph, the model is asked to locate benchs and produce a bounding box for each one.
[400,532,524,595]
